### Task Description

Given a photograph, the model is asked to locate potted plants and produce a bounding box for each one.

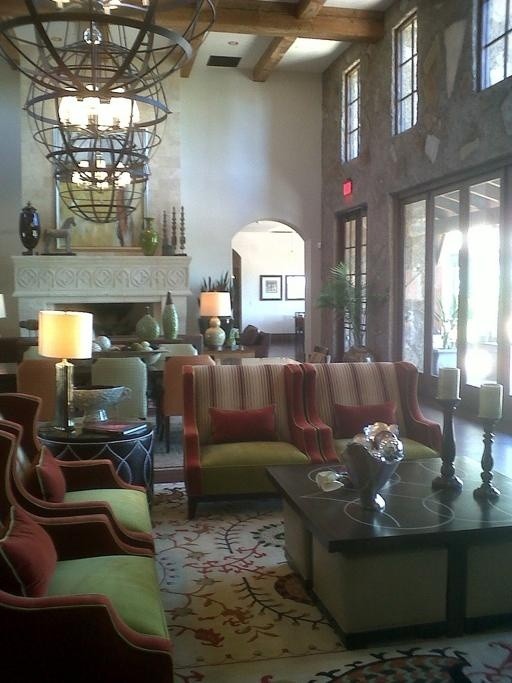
[315,260,389,362]
[432,295,457,376]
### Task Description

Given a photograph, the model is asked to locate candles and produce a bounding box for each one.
[476,381,503,417]
[439,366,460,398]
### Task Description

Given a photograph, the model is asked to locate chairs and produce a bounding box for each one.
[239,324,258,347]
[154,355,217,453]
[18,358,58,423]
[249,331,271,356]
[23,345,39,358]
[153,343,198,371]
[92,356,148,420]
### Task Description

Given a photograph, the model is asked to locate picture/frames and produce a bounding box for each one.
[285,274,305,300]
[259,274,282,300]
[56,178,146,254]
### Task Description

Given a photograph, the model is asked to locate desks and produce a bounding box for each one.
[200,346,302,364]
[18,331,203,431]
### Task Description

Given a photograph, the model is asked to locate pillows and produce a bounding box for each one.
[334,400,395,434]
[1,505,58,596]
[33,445,65,502]
[209,407,274,440]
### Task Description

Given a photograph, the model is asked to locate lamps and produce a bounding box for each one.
[23,39,170,189]
[1,0,215,94]
[55,125,148,222]
[199,291,231,350]
[39,310,93,431]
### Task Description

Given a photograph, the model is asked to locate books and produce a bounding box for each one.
[85,420,148,434]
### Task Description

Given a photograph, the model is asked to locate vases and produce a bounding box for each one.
[163,291,178,339]
[137,305,160,341]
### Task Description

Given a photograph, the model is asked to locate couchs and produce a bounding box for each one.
[182,362,325,521]
[1,430,173,682]
[0,393,153,549]
[303,362,442,458]
